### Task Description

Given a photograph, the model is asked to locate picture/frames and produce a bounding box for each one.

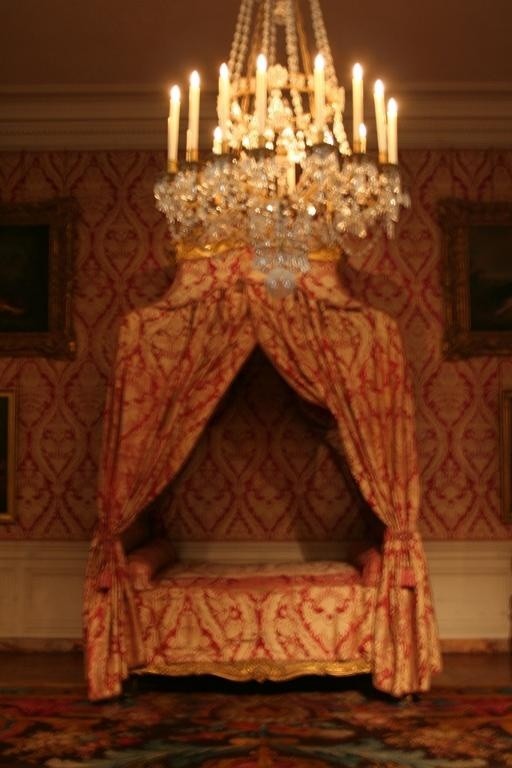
[0,196,79,360]
[0,388,17,524]
[498,389,512,523]
[443,199,512,358]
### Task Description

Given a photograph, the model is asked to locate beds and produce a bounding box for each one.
[84,241,445,706]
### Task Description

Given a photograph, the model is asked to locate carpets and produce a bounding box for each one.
[3,685,511,765]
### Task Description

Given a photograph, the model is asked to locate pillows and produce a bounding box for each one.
[99,540,178,592]
[359,549,419,590]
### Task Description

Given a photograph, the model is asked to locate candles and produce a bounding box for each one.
[166,53,399,175]
